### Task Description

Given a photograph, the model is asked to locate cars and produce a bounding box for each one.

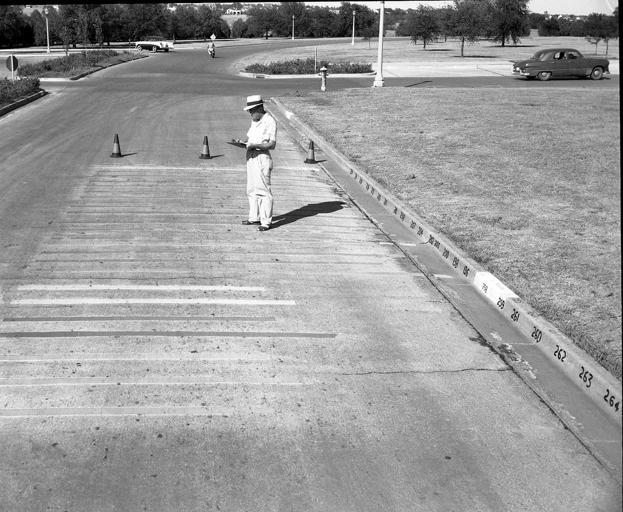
[510,48,611,81]
[262,30,272,36]
[133,35,174,52]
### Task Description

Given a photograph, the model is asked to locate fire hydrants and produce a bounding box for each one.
[318,65,329,92]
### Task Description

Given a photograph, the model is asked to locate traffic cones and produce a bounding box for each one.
[303,139,318,164]
[198,135,212,159]
[107,133,122,158]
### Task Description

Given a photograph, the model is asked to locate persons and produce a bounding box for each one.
[232,95,277,232]
[558,52,564,60]
[207,40,215,55]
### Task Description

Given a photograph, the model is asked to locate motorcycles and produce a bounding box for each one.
[208,50,214,58]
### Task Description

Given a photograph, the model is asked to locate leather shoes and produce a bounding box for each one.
[241,220,273,231]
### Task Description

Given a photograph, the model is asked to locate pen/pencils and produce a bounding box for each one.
[238,133,244,143]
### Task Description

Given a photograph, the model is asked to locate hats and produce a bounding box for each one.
[243,94,264,110]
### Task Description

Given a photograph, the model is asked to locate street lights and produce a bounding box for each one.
[350,10,356,46]
[44,7,50,54]
[290,14,296,40]
